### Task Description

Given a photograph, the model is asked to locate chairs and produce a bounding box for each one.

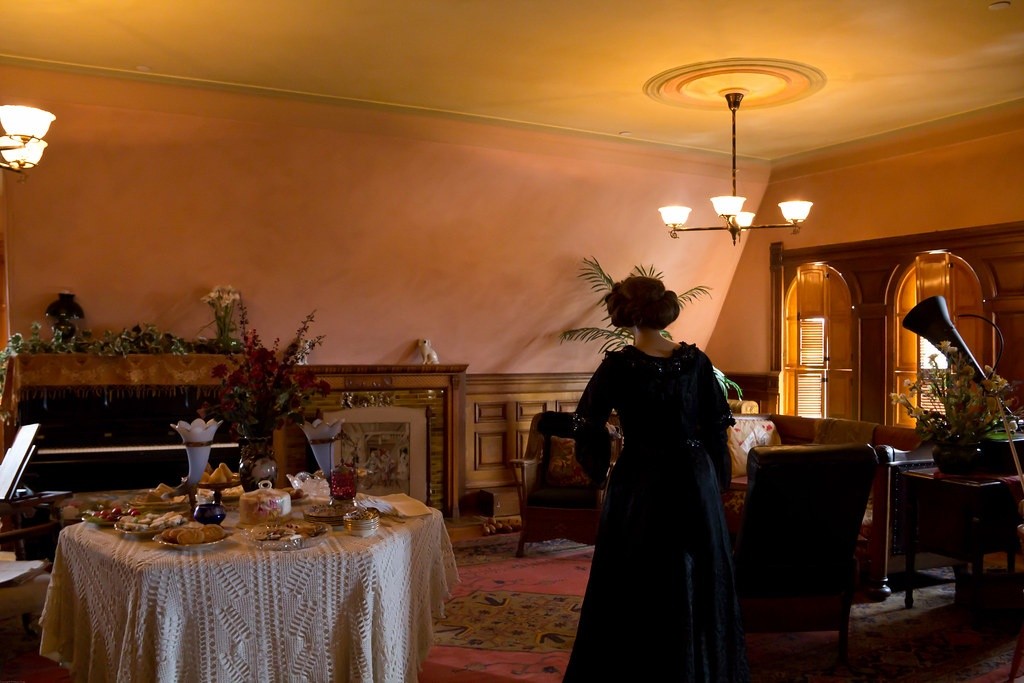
[508,411,621,557]
[734,440,880,666]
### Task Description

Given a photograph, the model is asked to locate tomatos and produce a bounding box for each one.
[92,508,140,521]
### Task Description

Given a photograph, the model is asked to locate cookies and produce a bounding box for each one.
[162,524,226,544]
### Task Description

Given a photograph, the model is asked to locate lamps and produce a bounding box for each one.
[0,104,56,172]
[45,292,84,339]
[657,87,814,247]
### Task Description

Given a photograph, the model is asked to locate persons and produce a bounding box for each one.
[561,277,751,683]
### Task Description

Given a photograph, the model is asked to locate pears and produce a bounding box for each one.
[201,463,232,483]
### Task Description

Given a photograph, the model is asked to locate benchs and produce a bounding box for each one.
[717,411,934,590]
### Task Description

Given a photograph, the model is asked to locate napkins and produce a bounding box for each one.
[355,492,432,518]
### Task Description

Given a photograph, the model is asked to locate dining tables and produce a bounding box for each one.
[37,483,463,683]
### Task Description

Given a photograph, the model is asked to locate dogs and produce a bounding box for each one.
[418,337,439,365]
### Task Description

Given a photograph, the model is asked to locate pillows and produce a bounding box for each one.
[543,435,593,489]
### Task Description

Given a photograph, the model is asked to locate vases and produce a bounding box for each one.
[238,431,277,493]
[206,335,242,354]
[170,418,224,487]
[931,436,985,476]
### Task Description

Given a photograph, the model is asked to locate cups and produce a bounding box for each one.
[331,466,358,501]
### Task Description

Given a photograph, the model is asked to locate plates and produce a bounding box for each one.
[152,530,233,549]
[113,521,163,538]
[129,495,186,510]
[304,505,362,526]
[81,515,116,526]
[239,521,333,550]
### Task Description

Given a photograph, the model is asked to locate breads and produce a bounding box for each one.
[146,483,174,501]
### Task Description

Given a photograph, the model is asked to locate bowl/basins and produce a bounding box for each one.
[342,513,380,537]
[286,470,330,497]
[194,503,226,525]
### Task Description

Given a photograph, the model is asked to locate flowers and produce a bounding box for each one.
[202,285,242,339]
[193,292,333,437]
[887,338,1018,442]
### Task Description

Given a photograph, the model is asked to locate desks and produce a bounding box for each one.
[900,465,1024,628]
